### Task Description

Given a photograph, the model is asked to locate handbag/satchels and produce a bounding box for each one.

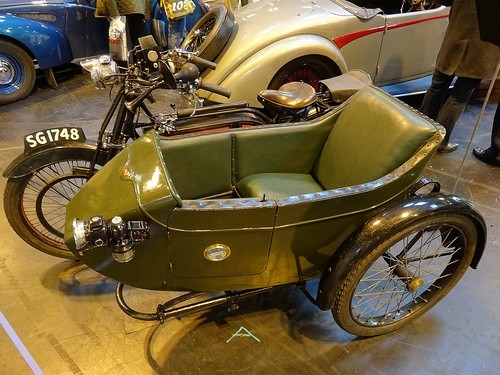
[108,16,127,62]
[159,0,196,21]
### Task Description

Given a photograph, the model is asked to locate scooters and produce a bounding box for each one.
[3,54,487,338]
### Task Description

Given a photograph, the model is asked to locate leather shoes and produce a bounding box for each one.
[436,143,459,154]
[473,147,500,167]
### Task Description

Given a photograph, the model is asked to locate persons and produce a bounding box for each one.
[95,0,151,65]
[419,0,500,155]
[472,100,499,167]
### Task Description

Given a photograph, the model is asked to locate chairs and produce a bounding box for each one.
[232,85,436,199]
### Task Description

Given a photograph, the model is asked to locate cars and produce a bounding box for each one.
[178,0,500,108]
[0,0,206,105]
